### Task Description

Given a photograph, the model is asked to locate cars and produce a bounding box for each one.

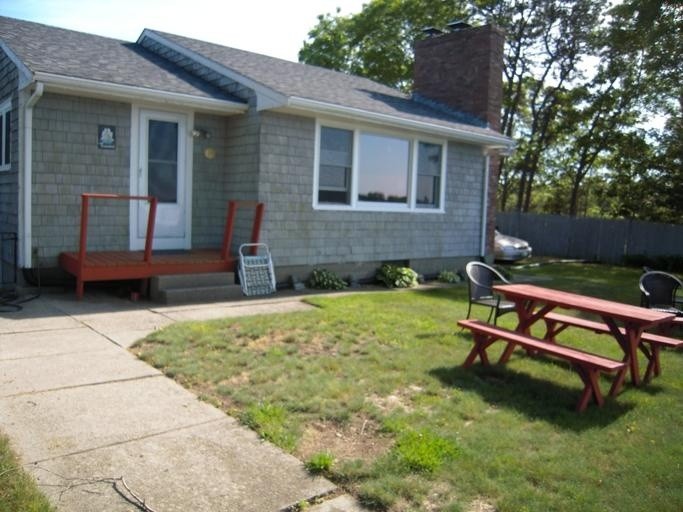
[494,228,533,261]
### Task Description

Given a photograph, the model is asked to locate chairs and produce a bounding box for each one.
[639,270,683,318]
[465,261,516,326]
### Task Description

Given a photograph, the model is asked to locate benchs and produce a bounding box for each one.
[457,319,630,414]
[530,311,683,383]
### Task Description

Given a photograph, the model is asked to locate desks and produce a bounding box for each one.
[494,284,676,398]
